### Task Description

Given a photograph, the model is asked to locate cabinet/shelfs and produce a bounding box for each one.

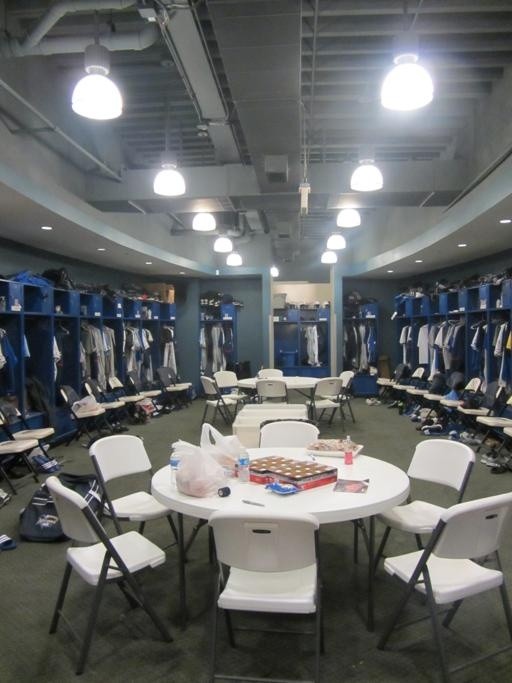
[346,271,512,469]
[270,305,335,377]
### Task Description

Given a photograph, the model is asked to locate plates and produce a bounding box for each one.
[302,443,363,458]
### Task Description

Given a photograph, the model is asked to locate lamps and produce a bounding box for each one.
[350,127,384,191]
[70,6,123,119]
[379,0,435,114]
[152,98,184,196]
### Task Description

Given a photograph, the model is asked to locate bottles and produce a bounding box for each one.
[170,448,180,483]
[345,434,354,464]
[239,445,249,482]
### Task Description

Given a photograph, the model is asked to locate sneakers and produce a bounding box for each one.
[365,397,383,406]
[387,395,512,476]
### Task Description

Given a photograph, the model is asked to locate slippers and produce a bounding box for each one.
[0,533,18,550]
[32,453,62,474]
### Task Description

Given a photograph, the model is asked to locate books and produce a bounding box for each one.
[234,453,338,484]
[235,471,336,492]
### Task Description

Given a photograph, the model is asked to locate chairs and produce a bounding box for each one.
[0,416,40,495]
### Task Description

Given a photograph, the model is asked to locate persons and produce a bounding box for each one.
[342,480,369,493]
